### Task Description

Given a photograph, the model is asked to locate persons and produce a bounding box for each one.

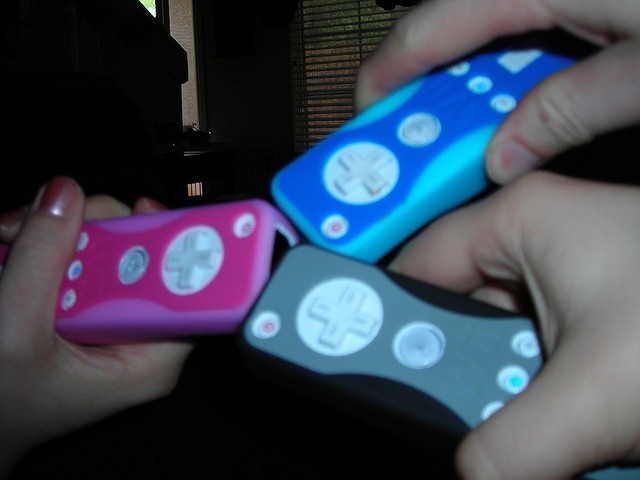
[0,176,195,480]
[387,170,639,480]
[355,1,639,187]
